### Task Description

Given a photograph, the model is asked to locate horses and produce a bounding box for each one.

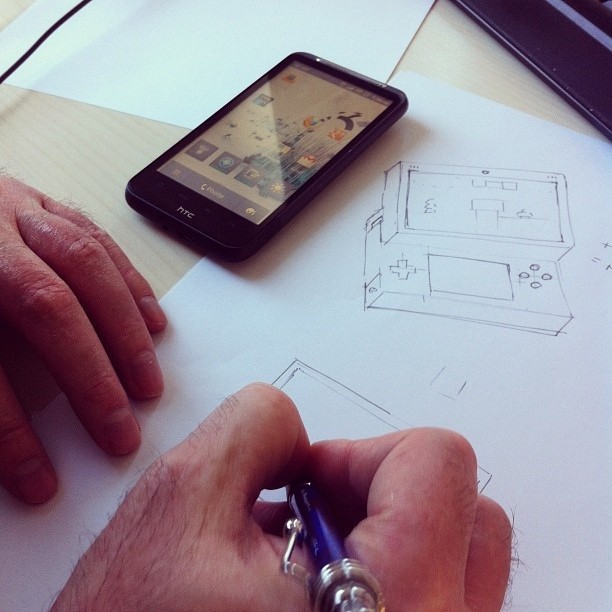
[125,50,409,260]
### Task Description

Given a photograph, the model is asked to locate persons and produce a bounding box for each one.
[0,172,513,611]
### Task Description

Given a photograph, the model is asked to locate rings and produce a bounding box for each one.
[282,485,387,612]
